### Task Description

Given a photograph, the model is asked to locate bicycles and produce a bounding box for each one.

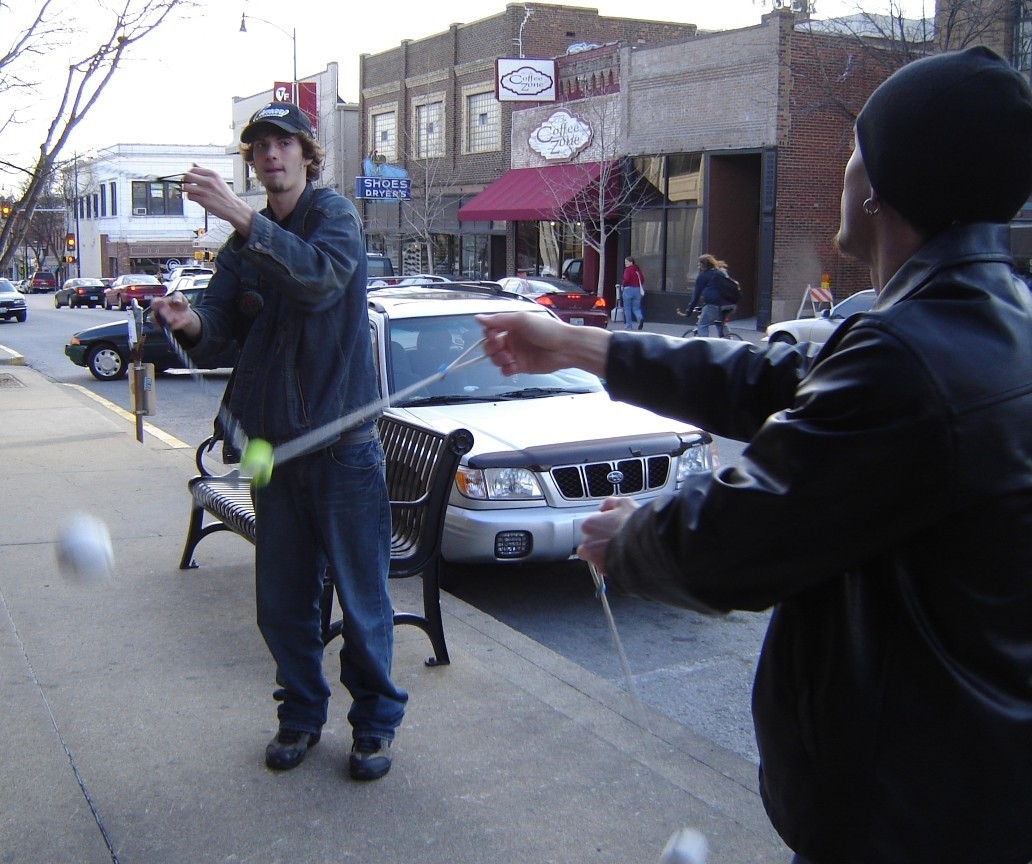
[674,304,746,341]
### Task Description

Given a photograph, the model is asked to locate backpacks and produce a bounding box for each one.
[710,268,740,304]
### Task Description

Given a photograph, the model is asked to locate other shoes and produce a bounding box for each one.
[638,319,644,330]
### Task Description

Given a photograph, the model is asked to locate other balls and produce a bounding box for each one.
[52,510,115,583]
[239,439,275,486]
[660,824,708,864]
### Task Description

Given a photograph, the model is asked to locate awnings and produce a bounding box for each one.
[456,159,621,221]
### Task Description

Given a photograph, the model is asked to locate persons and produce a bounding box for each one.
[471,44,1032,864]
[684,253,742,339]
[156,269,163,283]
[620,255,646,332]
[149,100,409,783]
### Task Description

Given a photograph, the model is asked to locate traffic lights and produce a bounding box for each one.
[68,256,75,263]
[1,199,10,220]
[192,228,205,239]
[67,231,75,251]
[204,251,214,260]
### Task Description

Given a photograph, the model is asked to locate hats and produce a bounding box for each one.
[854,46,1032,243]
[240,102,315,143]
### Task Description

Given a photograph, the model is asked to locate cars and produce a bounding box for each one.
[63,287,209,380]
[762,288,879,346]
[99,276,116,286]
[0,277,28,323]
[52,277,110,310]
[388,273,496,295]
[164,274,215,302]
[492,275,610,330]
[101,274,168,311]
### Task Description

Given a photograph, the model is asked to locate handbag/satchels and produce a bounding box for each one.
[637,272,644,295]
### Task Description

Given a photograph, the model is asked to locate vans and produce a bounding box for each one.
[367,252,395,287]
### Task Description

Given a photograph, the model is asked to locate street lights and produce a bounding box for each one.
[239,13,298,108]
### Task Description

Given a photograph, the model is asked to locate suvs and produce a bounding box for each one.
[363,277,720,567]
[162,265,215,292]
[8,270,56,294]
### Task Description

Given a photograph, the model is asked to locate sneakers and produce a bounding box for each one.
[349,737,392,781]
[265,729,322,771]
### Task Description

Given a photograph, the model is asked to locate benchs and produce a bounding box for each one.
[179,412,474,664]
